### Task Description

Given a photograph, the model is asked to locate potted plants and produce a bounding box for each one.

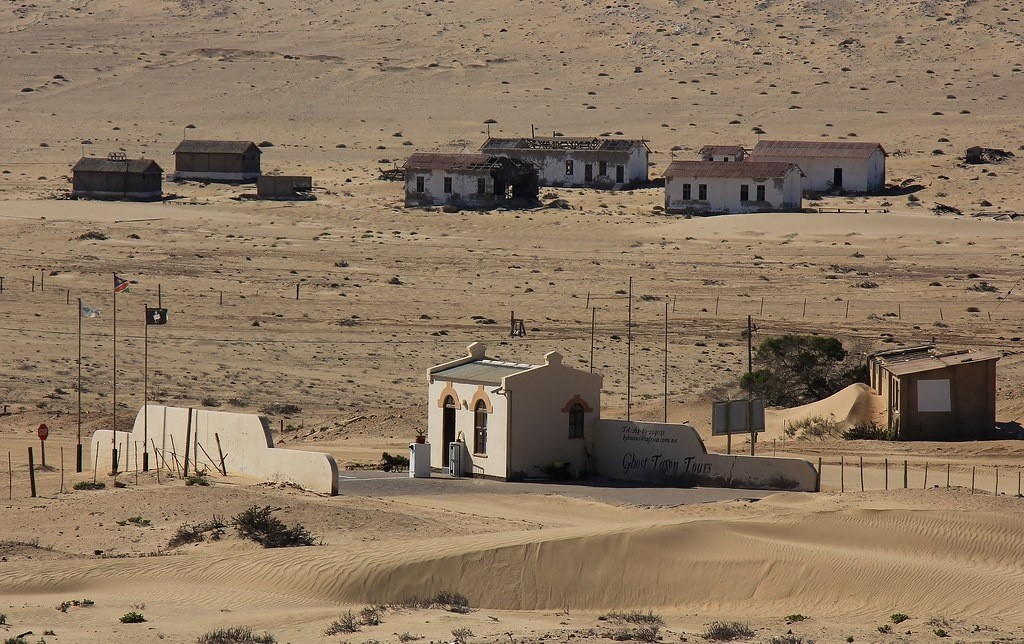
[415,429,426,444]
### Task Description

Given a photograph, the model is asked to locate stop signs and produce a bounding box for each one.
[38,423,49,441]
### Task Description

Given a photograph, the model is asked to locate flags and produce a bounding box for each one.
[113,276,131,294]
[145,308,168,325]
